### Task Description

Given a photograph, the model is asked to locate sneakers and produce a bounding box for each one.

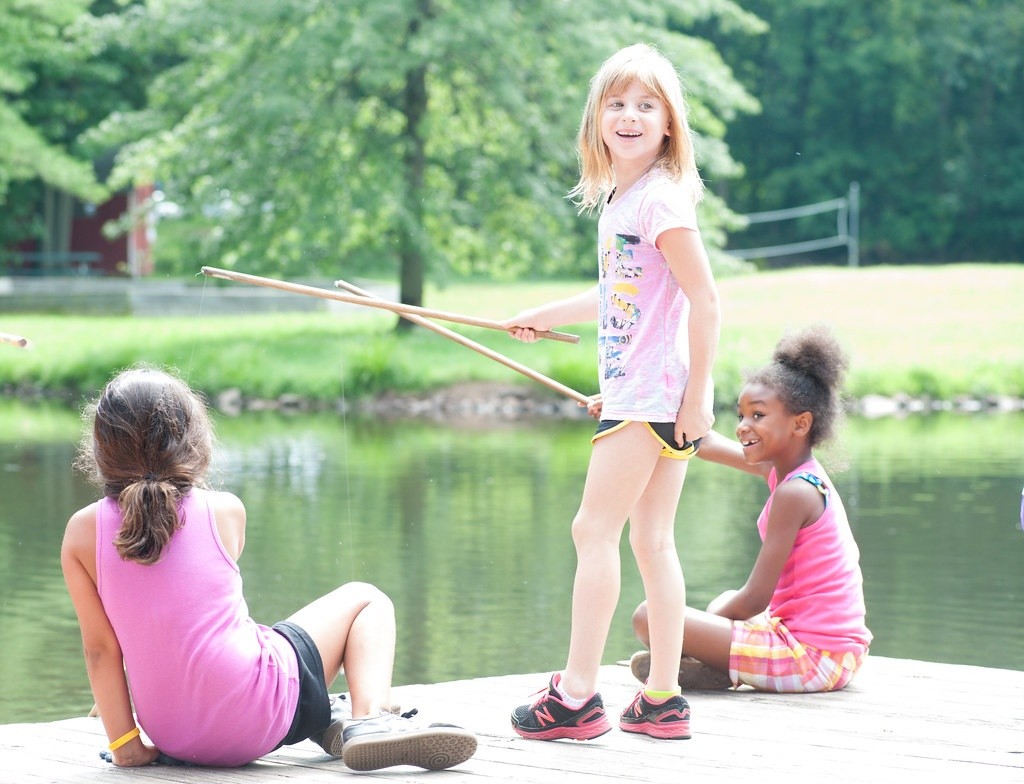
[511,671,613,741]
[619,677,693,740]
[309,694,401,756]
[341,708,479,772]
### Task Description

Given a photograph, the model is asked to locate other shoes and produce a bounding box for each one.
[629,651,734,691]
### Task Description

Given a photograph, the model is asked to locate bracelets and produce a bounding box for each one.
[109,727,140,754]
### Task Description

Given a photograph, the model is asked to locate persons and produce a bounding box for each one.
[575,325,876,690]
[494,42,723,745]
[61,364,479,772]
